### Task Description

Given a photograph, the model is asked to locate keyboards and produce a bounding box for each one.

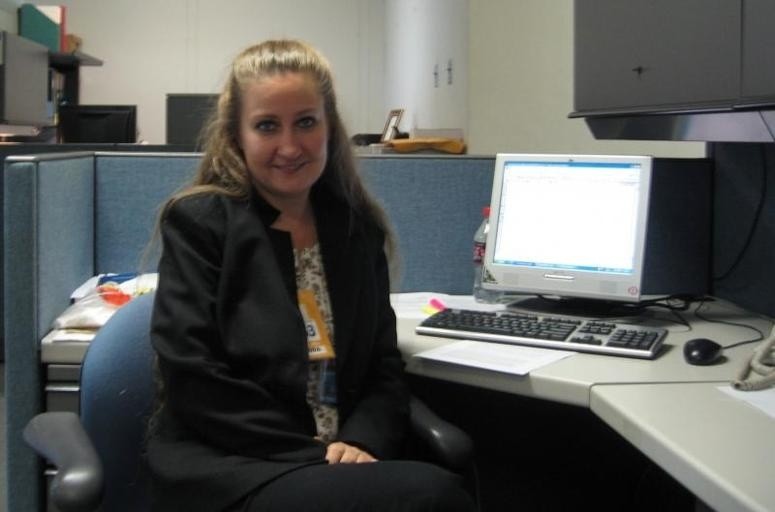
[415,308,668,360]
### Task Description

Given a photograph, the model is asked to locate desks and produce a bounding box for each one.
[590,382,774,512]
[40,280,775,408]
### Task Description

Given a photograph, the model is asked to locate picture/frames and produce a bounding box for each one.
[379,108,403,142]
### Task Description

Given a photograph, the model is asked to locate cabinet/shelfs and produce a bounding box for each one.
[408,2,471,136]
[0,30,104,131]
[572,0,775,118]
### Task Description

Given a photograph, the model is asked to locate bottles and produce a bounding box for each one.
[473,202,505,308]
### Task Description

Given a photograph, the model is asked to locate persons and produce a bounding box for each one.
[129,39,473,508]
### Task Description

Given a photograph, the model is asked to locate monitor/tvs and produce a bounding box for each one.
[56,104,136,144]
[480,152,653,317]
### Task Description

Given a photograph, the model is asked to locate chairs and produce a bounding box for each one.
[18,287,480,509]
[20,292,161,509]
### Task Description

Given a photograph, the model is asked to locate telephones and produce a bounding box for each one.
[751,325,775,376]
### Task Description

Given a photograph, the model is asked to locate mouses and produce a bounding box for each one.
[684,338,723,365]
